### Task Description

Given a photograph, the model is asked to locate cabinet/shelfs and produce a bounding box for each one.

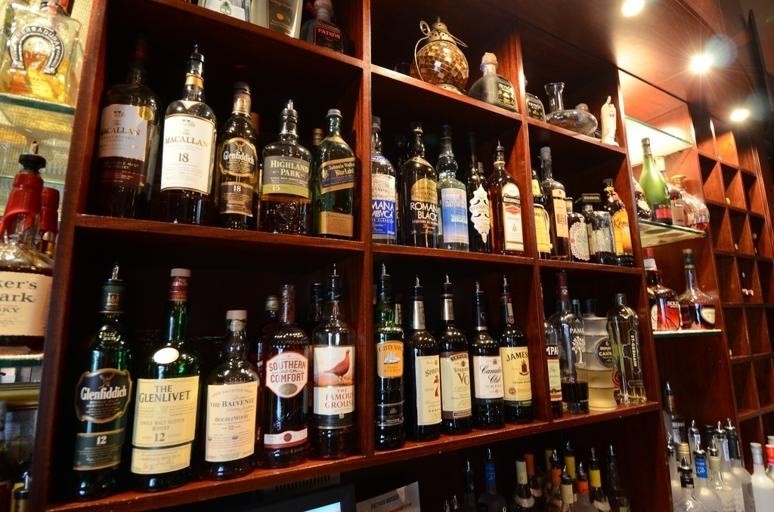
[619,68,774,510]
[33,0,674,512]
[0,0,99,512]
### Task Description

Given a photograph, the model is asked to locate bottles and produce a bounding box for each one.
[532,170,551,259]
[437,272,471,435]
[633,174,651,231]
[434,124,469,252]
[202,307,260,481]
[767,444,774,481]
[607,445,630,511]
[667,432,681,495]
[258,99,311,235]
[1,0,82,105]
[544,316,563,419]
[68,266,133,501]
[197,0,245,21]
[92,41,156,219]
[461,128,494,252]
[589,448,613,512]
[468,53,518,113]
[564,439,578,504]
[601,179,635,267]
[302,281,324,336]
[34,187,61,259]
[0,154,55,354]
[638,137,672,234]
[664,382,686,444]
[678,247,717,329]
[248,295,279,372]
[580,298,617,414]
[547,269,589,414]
[540,146,571,260]
[469,280,506,430]
[560,465,576,512]
[308,264,356,461]
[301,0,344,55]
[643,256,683,332]
[260,284,311,468]
[159,44,216,224]
[478,449,508,512]
[249,0,296,37]
[0,399,37,512]
[670,174,709,229]
[677,442,691,467]
[494,270,535,425]
[544,447,557,495]
[545,447,563,512]
[751,443,773,512]
[673,456,707,512]
[371,116,398,243]
[692,442,723,512]
[664,191,685,238]
[460,457,486,512]
[402,124,438,249]
[725,418,755,512]
[607,291,647,407]
[707,436,736,512]
[308,128,322,153]
[687,421,701,464]
[574,463,599,512]
[512,461,538,512]
[405,275,442,442]
[704,424,714,447]
[313,110,355,240]
[575,193,618,265]
[523,453,548,512]
[487,138,524,256]
[373,263,405,450]
[565,197,590,263]
[126,268,201,492]
[713,421,744,511]
[212,84,260,230]
